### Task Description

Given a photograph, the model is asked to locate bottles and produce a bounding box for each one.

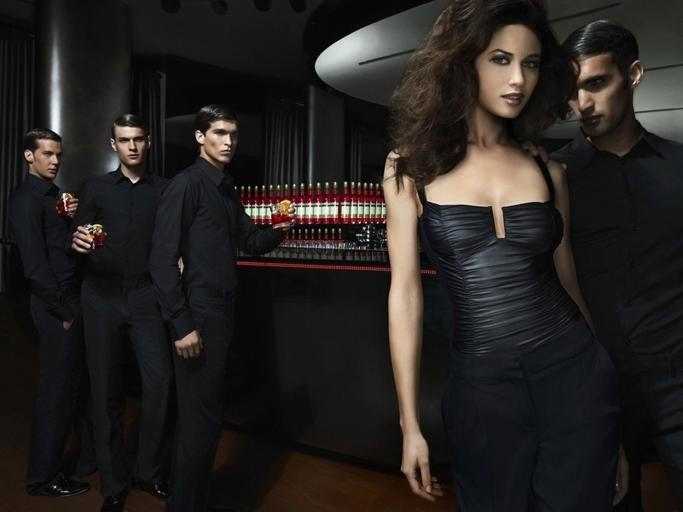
[233,180,389,249]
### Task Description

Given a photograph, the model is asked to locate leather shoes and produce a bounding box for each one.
[39,477,90,497]
[101,480,129,512]
[131,474,169,499]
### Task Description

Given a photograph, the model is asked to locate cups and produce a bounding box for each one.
[83,228,106,250]
[54,190,76,216]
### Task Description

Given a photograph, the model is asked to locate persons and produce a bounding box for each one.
[518,16,682,512]
[10,127,95,500]
[62,114,174,510]
[147,103,295,511]
[379,0,633,512]
